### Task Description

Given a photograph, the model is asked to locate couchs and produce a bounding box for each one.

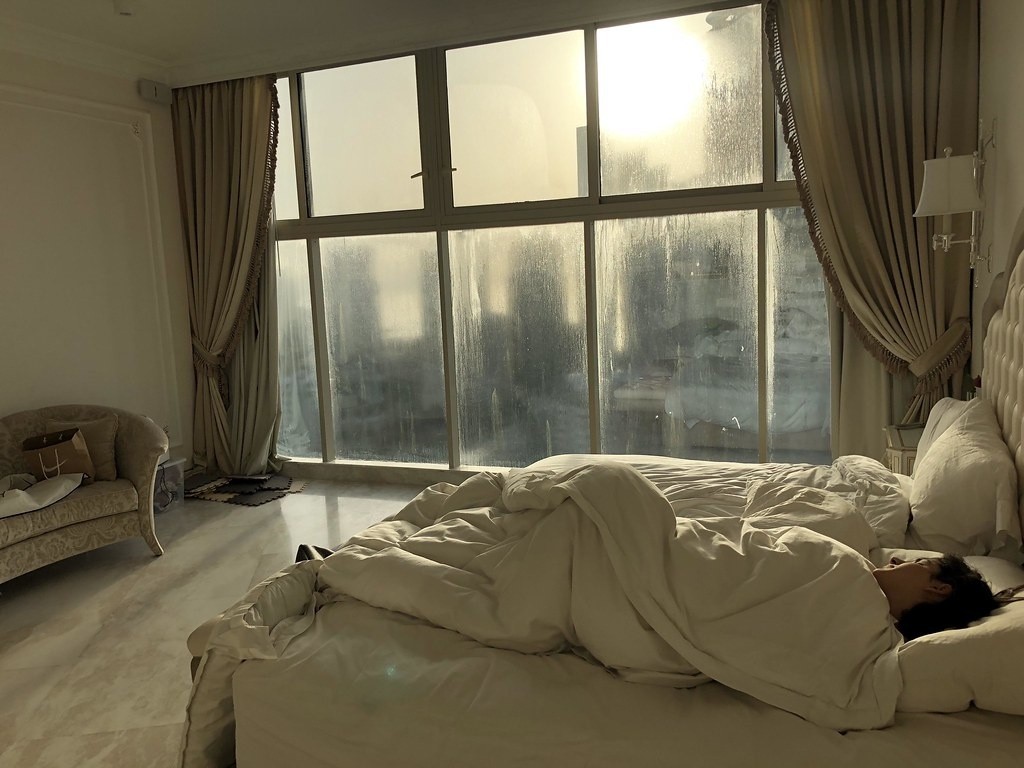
[0,404,169,586]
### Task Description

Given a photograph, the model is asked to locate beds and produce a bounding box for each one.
[661,289,832,454]
[177,206,1024,767]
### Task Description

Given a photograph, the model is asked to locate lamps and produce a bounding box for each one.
[912,116,996,289]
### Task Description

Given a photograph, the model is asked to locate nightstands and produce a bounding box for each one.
[884,423,923,477]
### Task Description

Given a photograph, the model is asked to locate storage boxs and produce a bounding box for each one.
[154,457,188,514]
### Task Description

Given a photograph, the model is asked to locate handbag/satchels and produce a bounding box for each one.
[22,431,96,483]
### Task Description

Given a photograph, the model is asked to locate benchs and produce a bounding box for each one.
[614,371,670,450]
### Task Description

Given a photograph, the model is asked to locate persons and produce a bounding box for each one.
[295,543,1024,644]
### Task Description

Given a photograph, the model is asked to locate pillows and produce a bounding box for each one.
[914,394,1021,554]
[870,547,1024,717]
[46,411,116,480]
